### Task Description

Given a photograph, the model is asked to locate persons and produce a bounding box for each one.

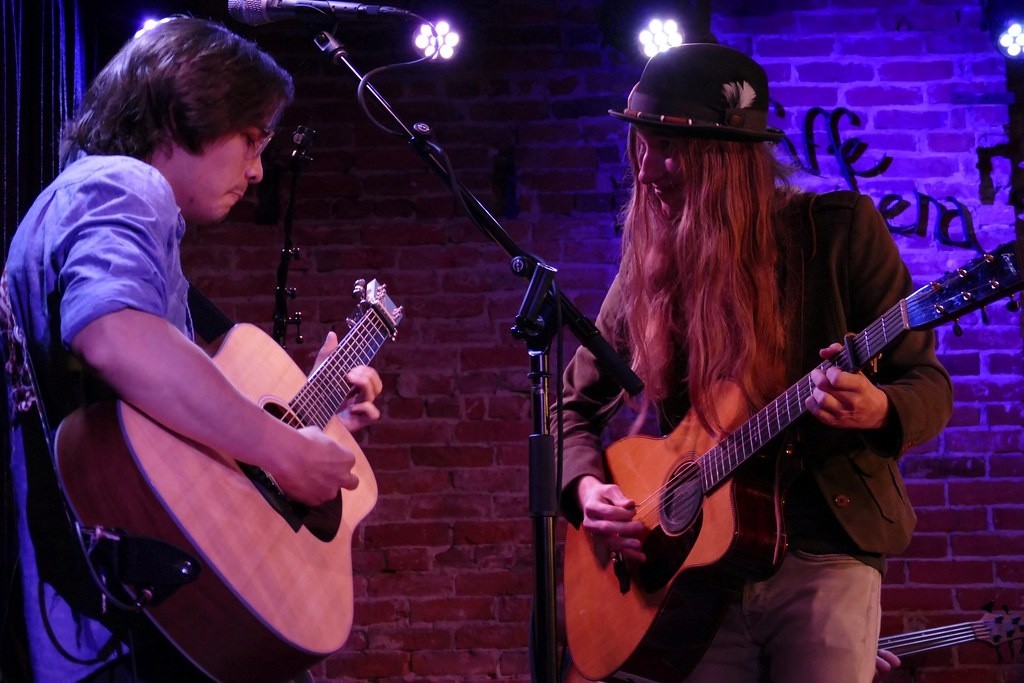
[0,19,383,683]
[550,44,953,683]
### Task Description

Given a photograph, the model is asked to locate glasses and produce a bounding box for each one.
[250,119,275,156]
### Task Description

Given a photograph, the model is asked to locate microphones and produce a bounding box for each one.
[228,0,412,27]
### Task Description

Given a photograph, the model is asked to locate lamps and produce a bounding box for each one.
[983,0,1024,61]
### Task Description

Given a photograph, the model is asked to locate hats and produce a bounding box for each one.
[606,41,785,142]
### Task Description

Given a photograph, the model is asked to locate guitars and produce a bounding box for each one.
[56,279,402,683]
[877,601,1023,664]
[562,239,1024,682]
